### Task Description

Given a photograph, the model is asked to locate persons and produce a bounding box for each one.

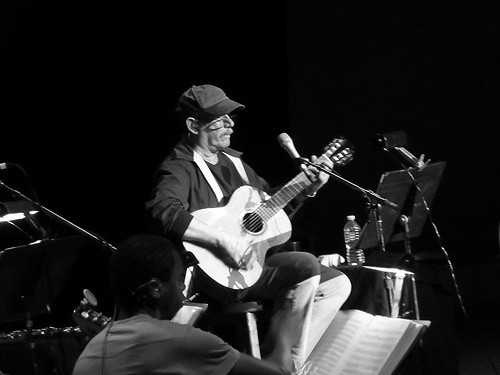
[71,234,303,375]
[142,83,352,375]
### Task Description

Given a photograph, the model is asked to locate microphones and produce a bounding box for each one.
[371,133,426,172]
[277,132,320,183]
[0,163,26,176]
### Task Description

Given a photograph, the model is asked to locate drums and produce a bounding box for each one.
[0,324,86,375]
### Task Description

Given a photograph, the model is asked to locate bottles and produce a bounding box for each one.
[343,215,365,266]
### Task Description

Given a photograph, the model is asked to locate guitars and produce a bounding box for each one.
[182,138,354,301]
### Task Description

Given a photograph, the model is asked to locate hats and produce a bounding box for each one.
[176,85,245,125]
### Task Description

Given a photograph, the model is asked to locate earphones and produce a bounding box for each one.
[150,280,159,292]
